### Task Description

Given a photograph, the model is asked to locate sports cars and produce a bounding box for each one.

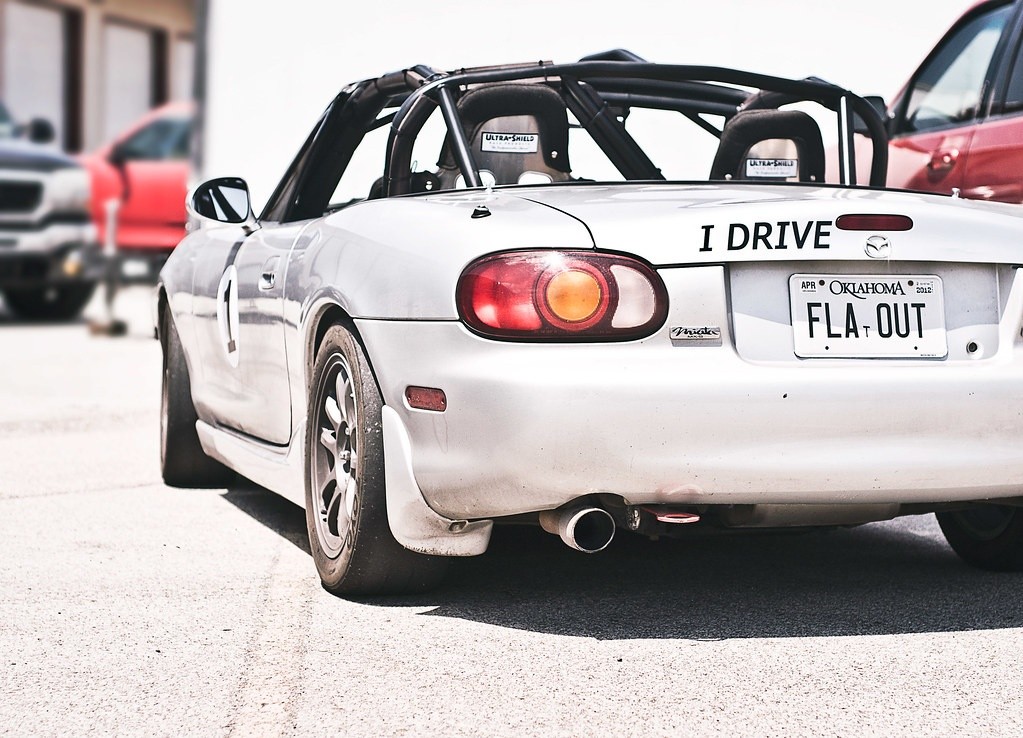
[153,49,1023,599]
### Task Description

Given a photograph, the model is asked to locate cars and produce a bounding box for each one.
[817,0,1023,204]
[82,101,196,278]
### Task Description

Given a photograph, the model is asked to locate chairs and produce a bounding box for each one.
[435,83,573,193]
[708,110,827,185]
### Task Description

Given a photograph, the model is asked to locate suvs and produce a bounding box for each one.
[0,105,103,322]
